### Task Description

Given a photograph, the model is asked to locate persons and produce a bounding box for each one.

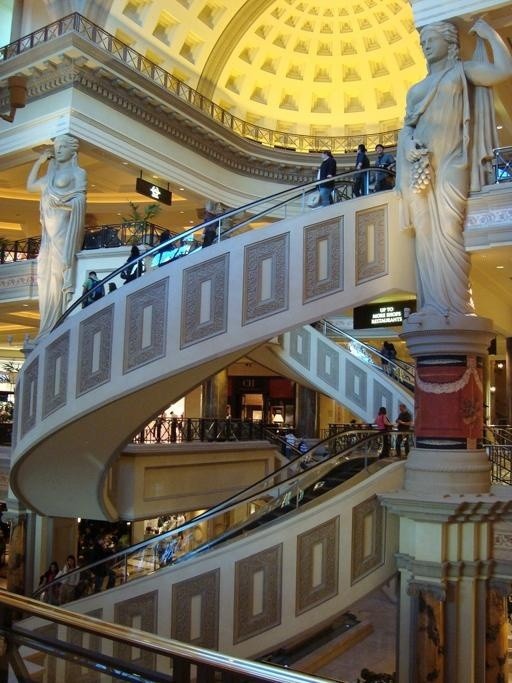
[108,282,117,294]
[81,271,105,309]
[38,521,122,607]
[158,230,176,267]
[393,404,414,460]
[25,133,88,339]
[144,514,186,567]
[375,407,395,459]
[373,144,396,191]
[393,10,512,325]
[379,340,398,377]
[200,210,222,249]
[315,151,336,207]
[120,245,142,284]
[351,144,370,198]
